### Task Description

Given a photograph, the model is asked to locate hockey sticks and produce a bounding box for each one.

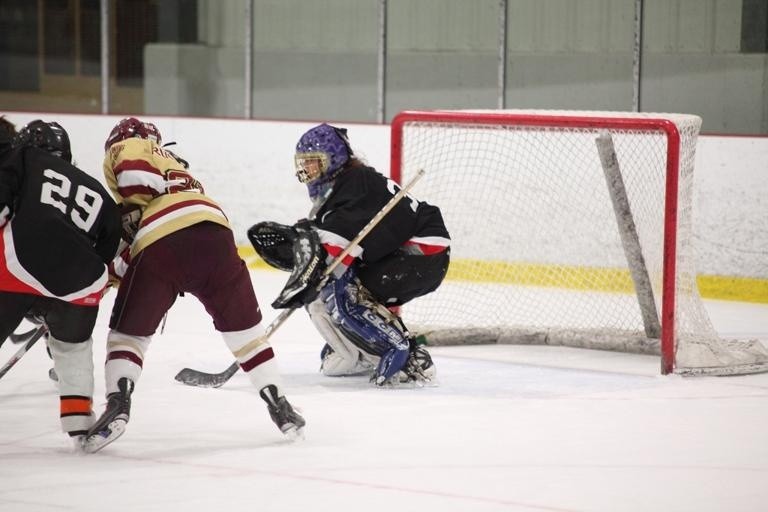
[8,328,37,345]
[175,170,425,390]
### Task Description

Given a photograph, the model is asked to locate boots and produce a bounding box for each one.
[260,385,305,435]
[80,376,134,441]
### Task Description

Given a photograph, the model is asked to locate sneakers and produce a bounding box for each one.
[324,352,373,376]
[374,339,413,386]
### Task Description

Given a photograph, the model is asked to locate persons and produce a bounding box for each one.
[0,119,123,438]
[0,116,17,155]
[245,123,451,386]
[72,118,304,444]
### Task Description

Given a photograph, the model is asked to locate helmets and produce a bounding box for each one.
[294,123,351,200]
[105,118,161,149]
[11,120,71,165]
